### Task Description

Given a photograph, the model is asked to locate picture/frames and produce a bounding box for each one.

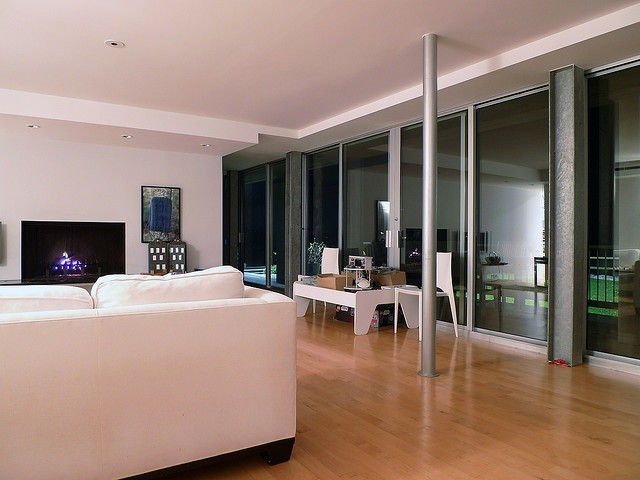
[140,184,181,245]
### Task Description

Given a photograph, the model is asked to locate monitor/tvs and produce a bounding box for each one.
[21,220,125,284]
[405,229,447,269]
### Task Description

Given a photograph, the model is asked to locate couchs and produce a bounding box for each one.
[0,283,296,479]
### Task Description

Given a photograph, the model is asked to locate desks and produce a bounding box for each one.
[293,280,420,336]
[1,273,153,285]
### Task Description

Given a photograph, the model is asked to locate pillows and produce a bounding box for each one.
[0,286,94,311]
[92,265,245,309]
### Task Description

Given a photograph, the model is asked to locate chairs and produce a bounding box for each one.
[394,252,458,339]
[475,253,503,332]
[300,247,340,314]
